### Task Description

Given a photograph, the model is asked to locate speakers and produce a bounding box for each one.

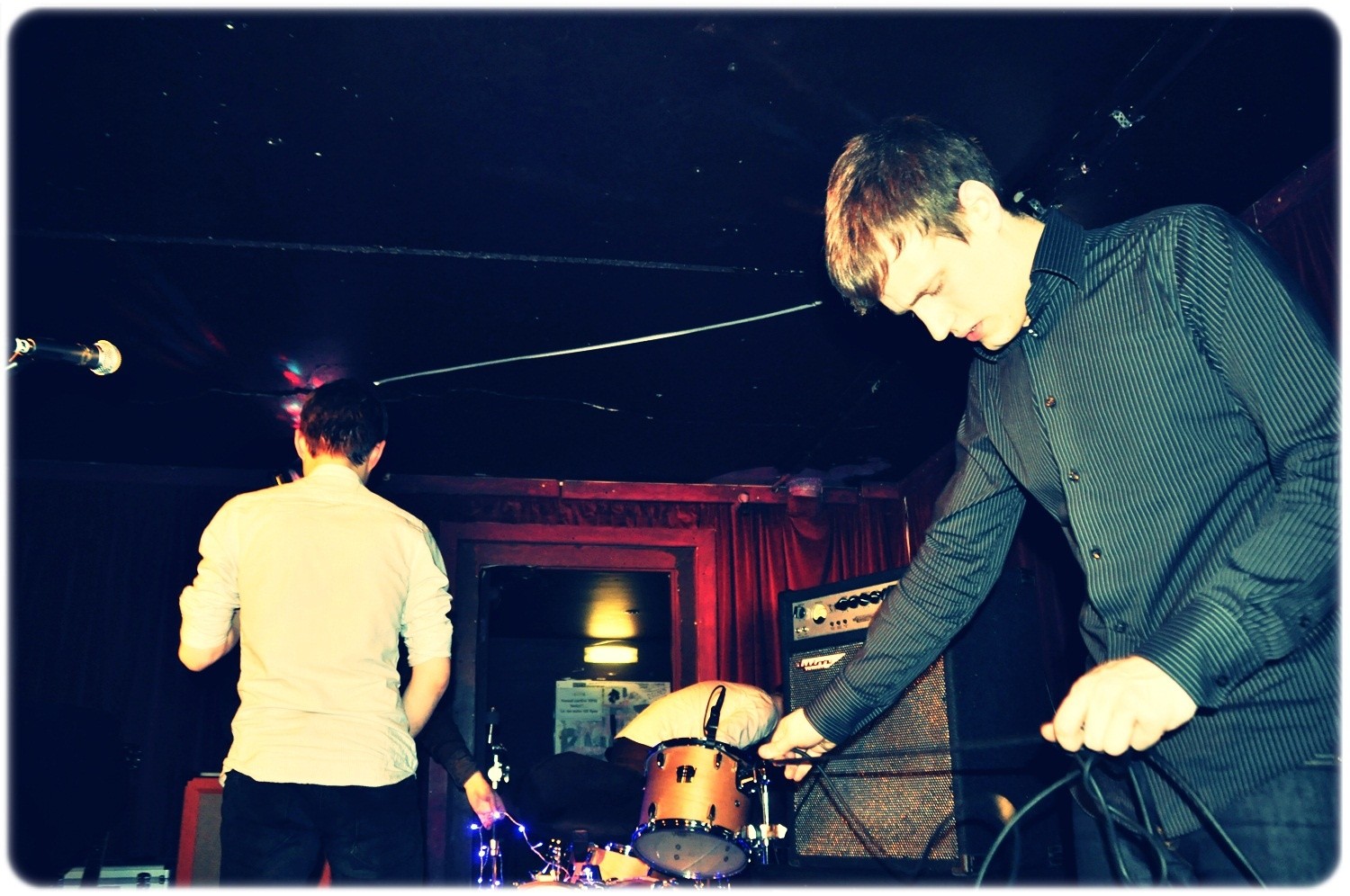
[177,777,332,888]
[782,630,1077,876]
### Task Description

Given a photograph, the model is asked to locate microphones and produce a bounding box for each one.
[706,687,726,731]
[11,337,121,375]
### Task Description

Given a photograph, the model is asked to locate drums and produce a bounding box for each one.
[630,736,754,881]
[563,840,652,885]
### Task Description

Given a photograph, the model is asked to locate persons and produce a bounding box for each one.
[177,376,453,885]
[398,658,506,830]
[760,115,1341,888]
